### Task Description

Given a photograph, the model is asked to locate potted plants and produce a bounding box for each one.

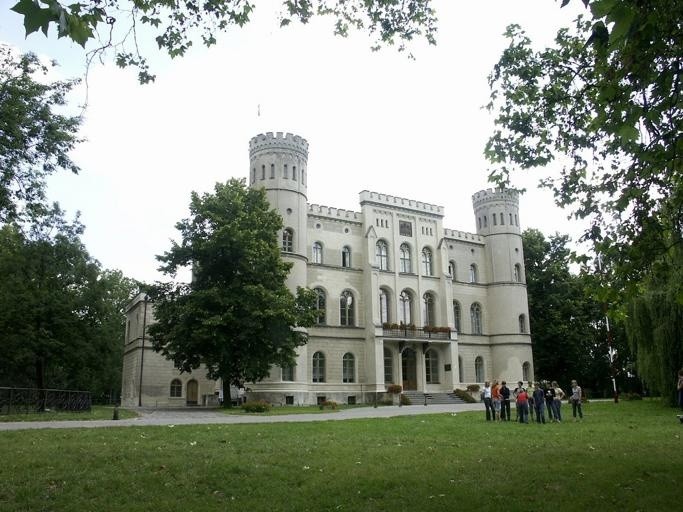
[388,384,402,405]
[465,384,481,401]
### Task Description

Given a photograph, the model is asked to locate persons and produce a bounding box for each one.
[569,379,583,423]
[482,380,496,421]
[512,379,566,423]
[490,379,504,421]
[675,368,683,424]
[499,380,511,421]
